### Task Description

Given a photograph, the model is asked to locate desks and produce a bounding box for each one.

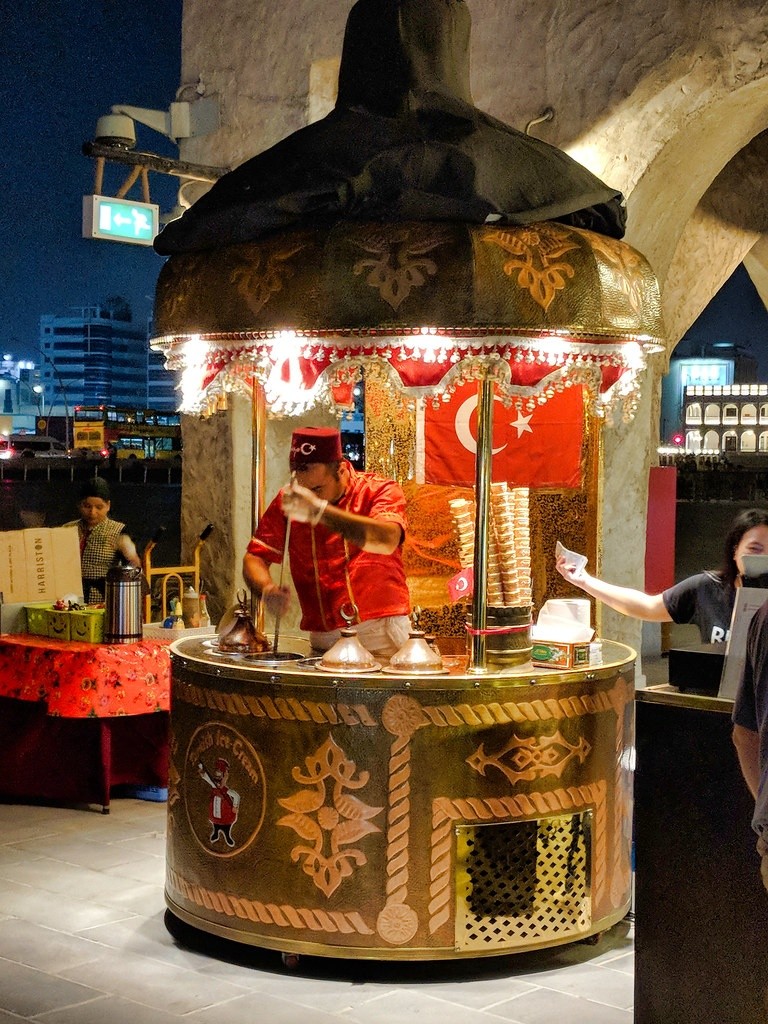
[631,681,767,1024]
[0,631,177,813]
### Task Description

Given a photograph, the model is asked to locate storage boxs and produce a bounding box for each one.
[141,622,217,648]
[23,604,104,643]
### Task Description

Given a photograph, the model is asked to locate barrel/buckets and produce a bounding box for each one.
[104,559,144,644]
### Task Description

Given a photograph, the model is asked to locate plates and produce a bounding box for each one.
[383,667,451,675]
[315,660,382,672]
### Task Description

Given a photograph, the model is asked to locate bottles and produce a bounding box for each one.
[199,594,210,627]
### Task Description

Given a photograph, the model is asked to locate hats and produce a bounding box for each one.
[79,477,110,501]
[288,427,343,462]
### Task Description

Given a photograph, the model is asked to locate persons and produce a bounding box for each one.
[729,596,768,892]
[554,507,768,645]
[241,425,414,656]
[57,477,142,605]
